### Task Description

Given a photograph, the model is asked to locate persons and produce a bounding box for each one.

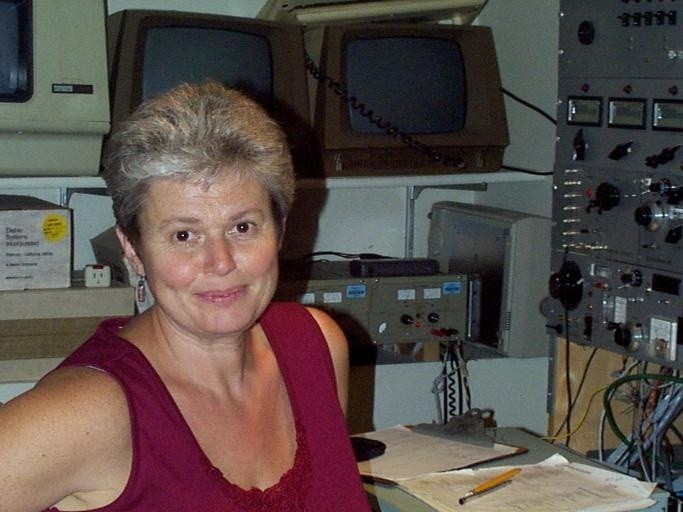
[0,83,370,512]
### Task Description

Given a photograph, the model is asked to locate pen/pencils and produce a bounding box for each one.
[459,468,522,504]
[358,471,400,487]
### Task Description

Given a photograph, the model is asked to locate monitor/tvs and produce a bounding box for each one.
[105,9,308,171]
[314,24,510,176]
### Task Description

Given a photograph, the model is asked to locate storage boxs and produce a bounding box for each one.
[0,194,71,291]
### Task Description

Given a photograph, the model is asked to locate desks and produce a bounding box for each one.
[364,427,671,512]
